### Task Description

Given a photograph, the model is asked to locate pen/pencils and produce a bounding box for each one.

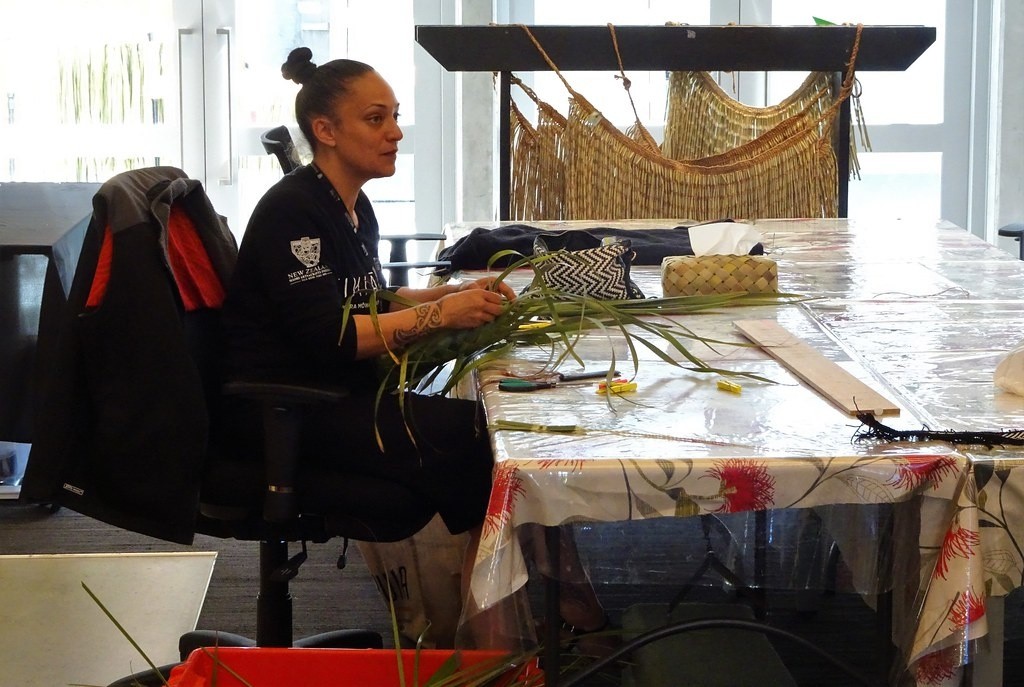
[559,371,622,382]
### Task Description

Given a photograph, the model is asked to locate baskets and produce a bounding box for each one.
[661,253,779,297]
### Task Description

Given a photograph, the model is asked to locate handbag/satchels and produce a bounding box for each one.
[16,201,215,545]
[518,229,646,301]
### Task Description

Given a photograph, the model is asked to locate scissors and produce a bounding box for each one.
[498,379,593,391]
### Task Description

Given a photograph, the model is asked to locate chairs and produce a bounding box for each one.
[262,125,453,284]
[91,164,386,686]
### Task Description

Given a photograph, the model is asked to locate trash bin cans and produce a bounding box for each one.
[161,646,545,687]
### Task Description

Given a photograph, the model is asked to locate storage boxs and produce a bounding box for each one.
[164,645,546,687]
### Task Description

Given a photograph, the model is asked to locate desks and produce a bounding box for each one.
[439,213,1024,687]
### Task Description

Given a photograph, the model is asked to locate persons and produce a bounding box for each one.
[216,47,633,671]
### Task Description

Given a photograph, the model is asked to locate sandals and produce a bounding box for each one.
[556,612,634,672]
